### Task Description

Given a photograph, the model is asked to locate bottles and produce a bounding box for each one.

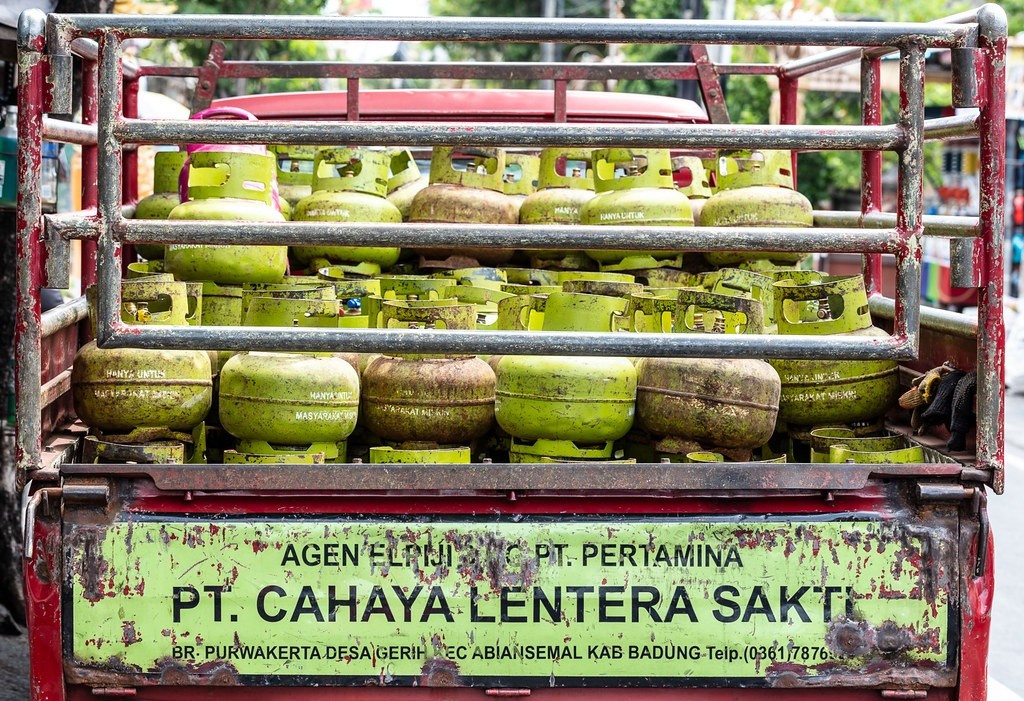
[1011,266,1020,299]
[1013,227,1024,263]
[0,105,59,201]
[1015,189,1024,225]
[928,201,968,216]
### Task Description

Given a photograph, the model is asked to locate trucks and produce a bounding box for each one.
[14,2,1006,701]
